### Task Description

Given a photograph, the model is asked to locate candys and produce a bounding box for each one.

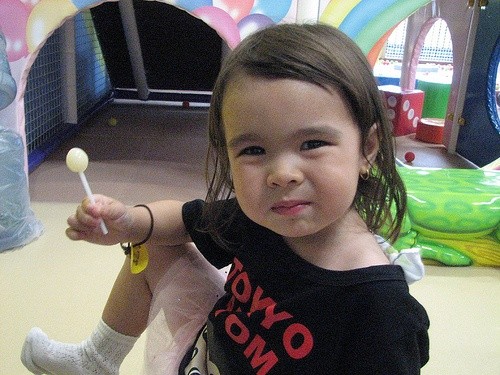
[66,147,108,234]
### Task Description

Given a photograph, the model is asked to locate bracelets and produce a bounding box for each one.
[120,204,154,255]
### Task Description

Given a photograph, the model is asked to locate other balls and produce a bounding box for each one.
[405,151,416,163]
[106,117,118,127]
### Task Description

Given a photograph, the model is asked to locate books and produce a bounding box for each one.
[19,23,430,375]
